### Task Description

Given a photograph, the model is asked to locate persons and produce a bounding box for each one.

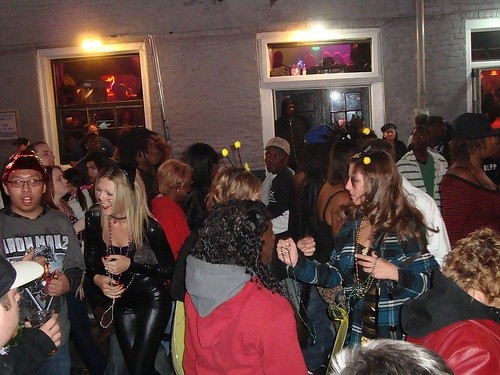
[325,337,454,375]
[0,253,62,375]
[354,206,383,298]
[0,95,500,375]
[181,198,308,375]
[400,228,500,375]
[76,163,176,375]
[274,148,437,374]
[0,152,87,375]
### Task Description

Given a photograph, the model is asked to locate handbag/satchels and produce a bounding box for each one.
[134,243,159,266]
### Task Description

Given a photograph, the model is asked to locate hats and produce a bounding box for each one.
[0,252,45,298]
[452,112,499,139]
[262,136,290,156]
[304,123,336,142]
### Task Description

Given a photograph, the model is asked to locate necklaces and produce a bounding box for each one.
[113,219,118,225]
[99,212,131,330]
[279,246,318,346]
[364,255,380,295]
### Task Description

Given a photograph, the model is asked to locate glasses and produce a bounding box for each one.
[7,177,44,187]
